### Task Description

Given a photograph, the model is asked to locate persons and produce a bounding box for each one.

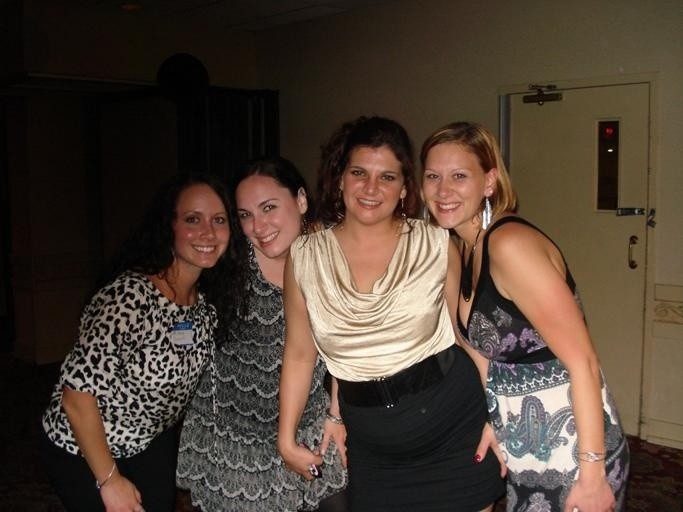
[414,119,633,512]
[33,167,245,512]
[276,112,512,512]
[174,151,351,512]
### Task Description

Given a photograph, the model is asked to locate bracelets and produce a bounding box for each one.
[323,408,344,426]
[570,446,607,465]
[95,461,117,490]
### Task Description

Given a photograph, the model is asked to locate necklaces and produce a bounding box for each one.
[459,225,484,303]
